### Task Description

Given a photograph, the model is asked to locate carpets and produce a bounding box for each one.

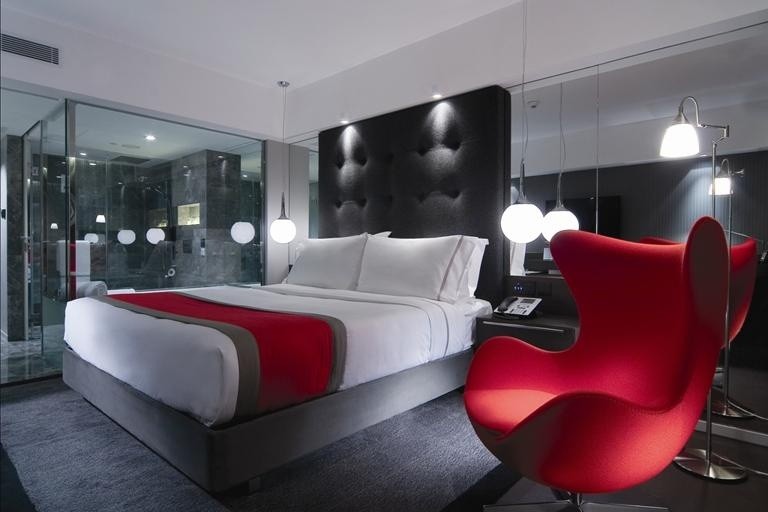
[0,376,501,512]
[487,463,768,512]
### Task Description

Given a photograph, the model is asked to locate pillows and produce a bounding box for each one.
[358,235,474,301]
[459,238,489,299]
[281,232,390,288]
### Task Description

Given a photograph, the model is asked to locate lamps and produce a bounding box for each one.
[500,0,543,244]
[118,229,135,244]
[709,160,753,418]
[231,223,255,243]
[272,81,295,244]
[660,96,744,481]
[146,228,164,245]
[85,234,99,244]
[542,84,579,242]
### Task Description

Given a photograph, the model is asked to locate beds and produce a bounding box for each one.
[62,284,493,492]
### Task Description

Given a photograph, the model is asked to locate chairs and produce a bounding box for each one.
[464,214,730,512]
[645,230,755,350]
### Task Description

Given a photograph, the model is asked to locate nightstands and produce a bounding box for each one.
[476,317,575,352]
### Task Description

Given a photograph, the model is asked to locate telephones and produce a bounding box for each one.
[493,295,543,322]
